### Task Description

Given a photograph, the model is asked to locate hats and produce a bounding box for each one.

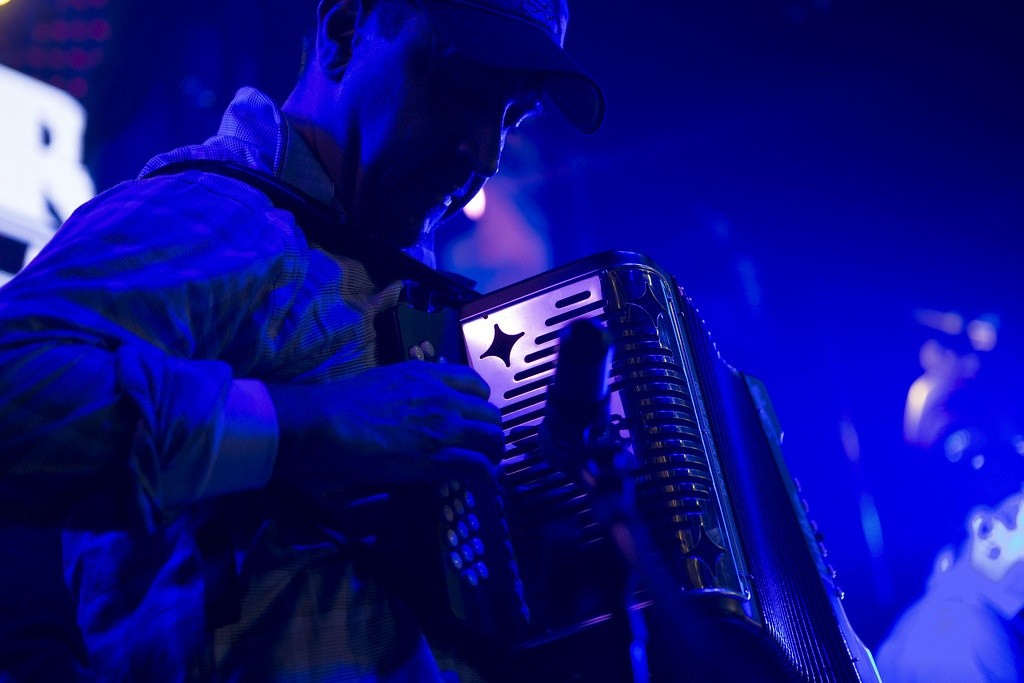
[416,0,605,136]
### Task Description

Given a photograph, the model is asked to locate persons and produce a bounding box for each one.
[873,436,1024,683]
[0,0,604,683]
